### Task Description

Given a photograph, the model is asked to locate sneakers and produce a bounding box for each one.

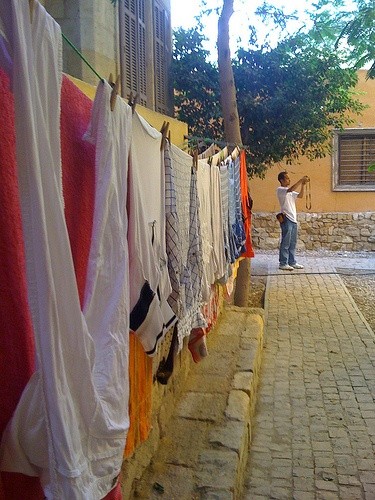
[279,264,293,271]
[293,263,305,269]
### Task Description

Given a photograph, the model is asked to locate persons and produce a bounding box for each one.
[275,171,311,271]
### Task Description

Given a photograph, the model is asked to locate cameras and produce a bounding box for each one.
[304,176,310,182]
